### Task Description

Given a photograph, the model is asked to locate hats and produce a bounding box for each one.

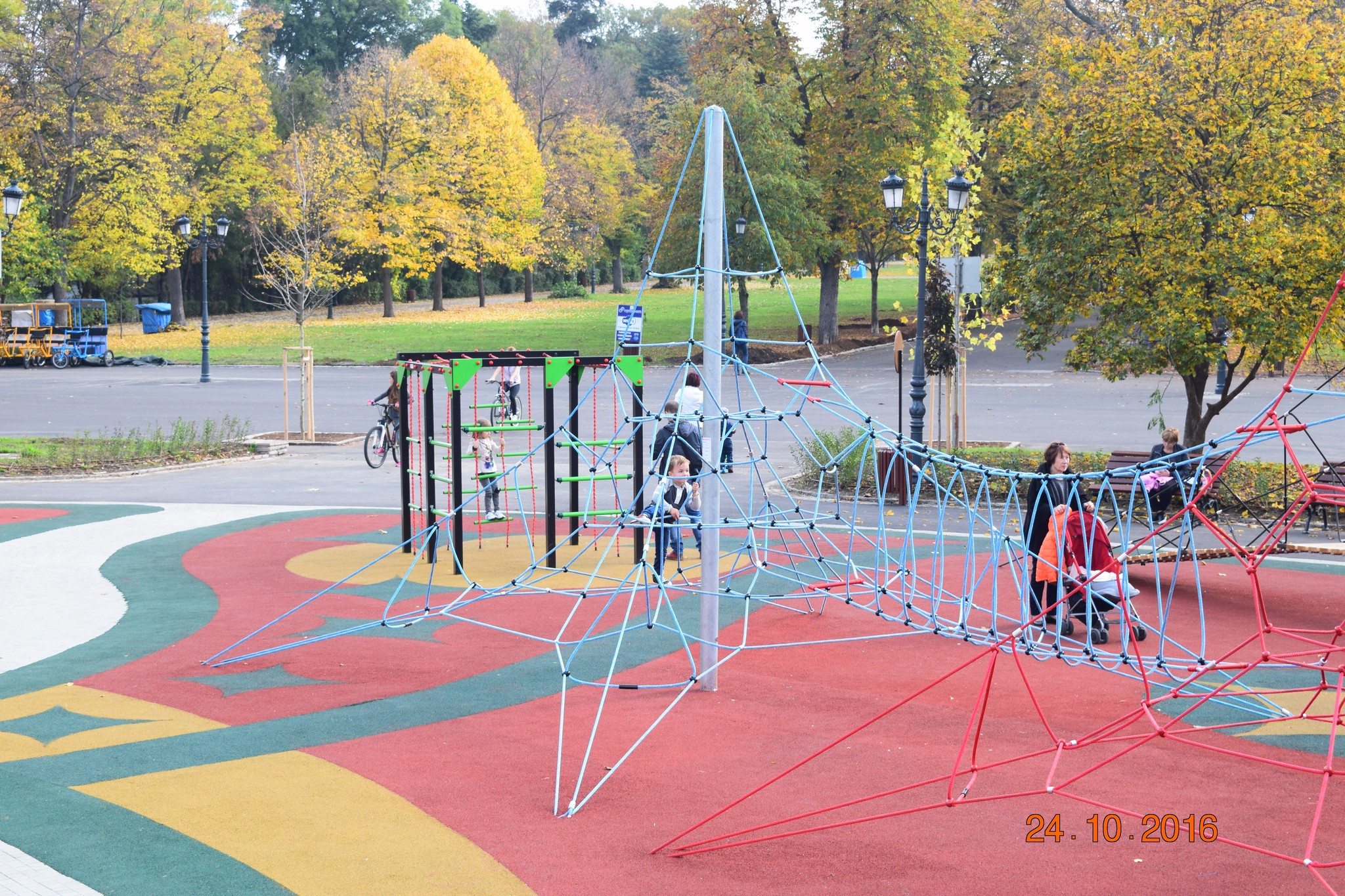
[720,407,729,415]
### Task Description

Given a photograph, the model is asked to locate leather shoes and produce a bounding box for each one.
[1045,615,1056,624]
[1031,615,1043,626]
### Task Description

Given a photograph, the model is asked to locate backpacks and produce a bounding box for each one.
[1195,466,1213,491]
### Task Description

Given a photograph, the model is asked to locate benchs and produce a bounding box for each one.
[1299,461,1345,534]
[1089,451,1229,522]
[6,327,108,345]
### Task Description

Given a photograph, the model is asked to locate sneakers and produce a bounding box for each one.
[652,573,672,586]
[630,514,650,524]
[495,511,505,519]
[666,550,683,561]
[396,462,401,467]
[374,447,385,455]
[486,511,497,520]
[700,553,701,559]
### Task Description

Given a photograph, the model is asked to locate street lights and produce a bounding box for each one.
[176,214,230,383]
[881,162,975,480]
[1215,204,1257,392]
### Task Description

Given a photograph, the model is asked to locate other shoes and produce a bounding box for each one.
[512,415,517,421]
[720,466,725,470]
[742,371,749,374]
[728,465,733,473]
[499,406,506,412]
[1158,513,1167,525]
[737,374,740,375]
[1147,513,1158,523]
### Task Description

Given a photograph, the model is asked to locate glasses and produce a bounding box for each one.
[1049,442,1066,449]
[1162,439,1175,445]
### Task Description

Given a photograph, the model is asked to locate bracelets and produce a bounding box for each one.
[472,445,478,451]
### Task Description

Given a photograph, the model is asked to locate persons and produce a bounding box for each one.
[630,454,701,586]
[367,371,415,466]
[466,419,507,520]
[728,310,751,375]
[486,346,522,421]
[1023,441,1097,626]
[649,370,736,560]
[1139,427,1192,524]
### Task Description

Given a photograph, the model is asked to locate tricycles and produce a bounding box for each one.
[0,297,115,368]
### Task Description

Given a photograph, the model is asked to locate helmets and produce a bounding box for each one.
[1181,474,1203,490]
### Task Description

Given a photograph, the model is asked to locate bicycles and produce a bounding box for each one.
[363,402,411,468]
[485,379,522,434]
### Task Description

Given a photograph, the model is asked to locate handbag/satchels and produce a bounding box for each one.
[735,321,748,346]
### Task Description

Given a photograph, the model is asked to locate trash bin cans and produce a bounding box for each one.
[850,260,866,278]
[34,300,55,326]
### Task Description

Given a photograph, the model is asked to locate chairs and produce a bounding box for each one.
[26,327,53,355]
[64,329,89,355]
[0,327,17,355]
